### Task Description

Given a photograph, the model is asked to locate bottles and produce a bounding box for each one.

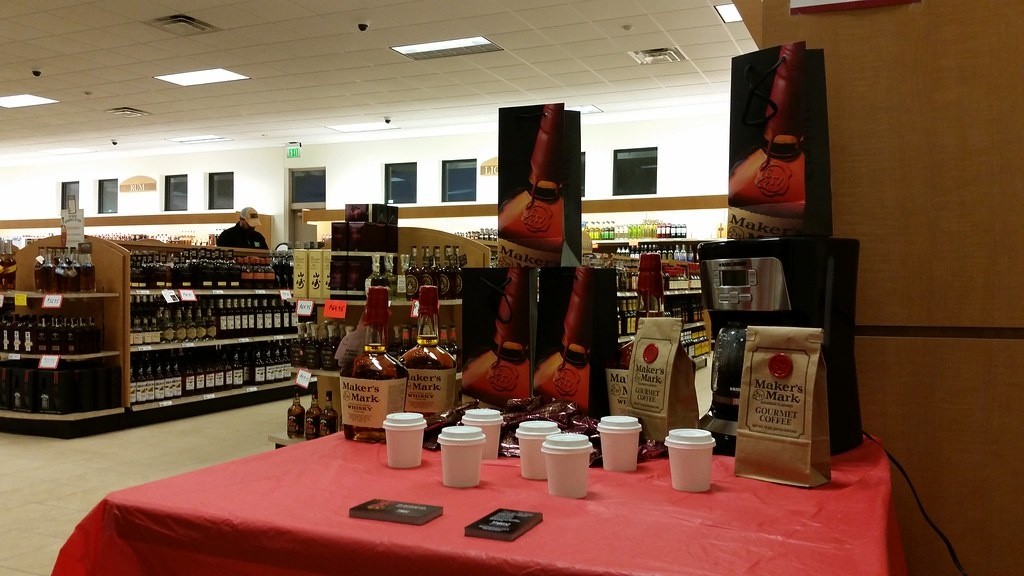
[365,245,461,301]
[128,247,294,290]
[498,103,565,267]
[130,294,318,346]
[728,42,805,239]
[581,220,709,368]
[0,240,16,292]
[61,217,67,247]
[95,233,218,248]
[130,338,299,405]
[292,321,457,371]
[339,286,457,452]
[454,228,498,242]
[288,390,342,441]
[34,243,96,295]
[617,253,668,370]
[460,267,591,409]
[0,313,101,355]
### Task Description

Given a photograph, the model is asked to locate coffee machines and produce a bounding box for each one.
[698,237,864,457]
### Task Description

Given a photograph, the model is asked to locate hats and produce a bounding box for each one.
[240,207,261,227]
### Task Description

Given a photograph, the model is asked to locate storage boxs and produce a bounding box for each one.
[29,365,121,415]
[0,367,29,413]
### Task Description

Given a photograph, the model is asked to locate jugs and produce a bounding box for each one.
[709,322,747,404]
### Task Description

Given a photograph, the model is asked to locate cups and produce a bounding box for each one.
[596,415,642,471]
[461,409,503,459]
[437,426,487,487]
[540,434,594,499]
[664,428,717,492]
[514,421,561,480]
[382,412,428,468]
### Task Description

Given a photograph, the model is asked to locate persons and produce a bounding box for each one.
[216,207,269,249]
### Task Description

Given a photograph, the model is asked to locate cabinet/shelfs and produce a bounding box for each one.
[0,227,711,440]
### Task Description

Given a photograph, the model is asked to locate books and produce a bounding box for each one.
[349,498,443,525]
[464,508,544,542]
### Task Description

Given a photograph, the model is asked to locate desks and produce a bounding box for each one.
[50,430,909,576]
